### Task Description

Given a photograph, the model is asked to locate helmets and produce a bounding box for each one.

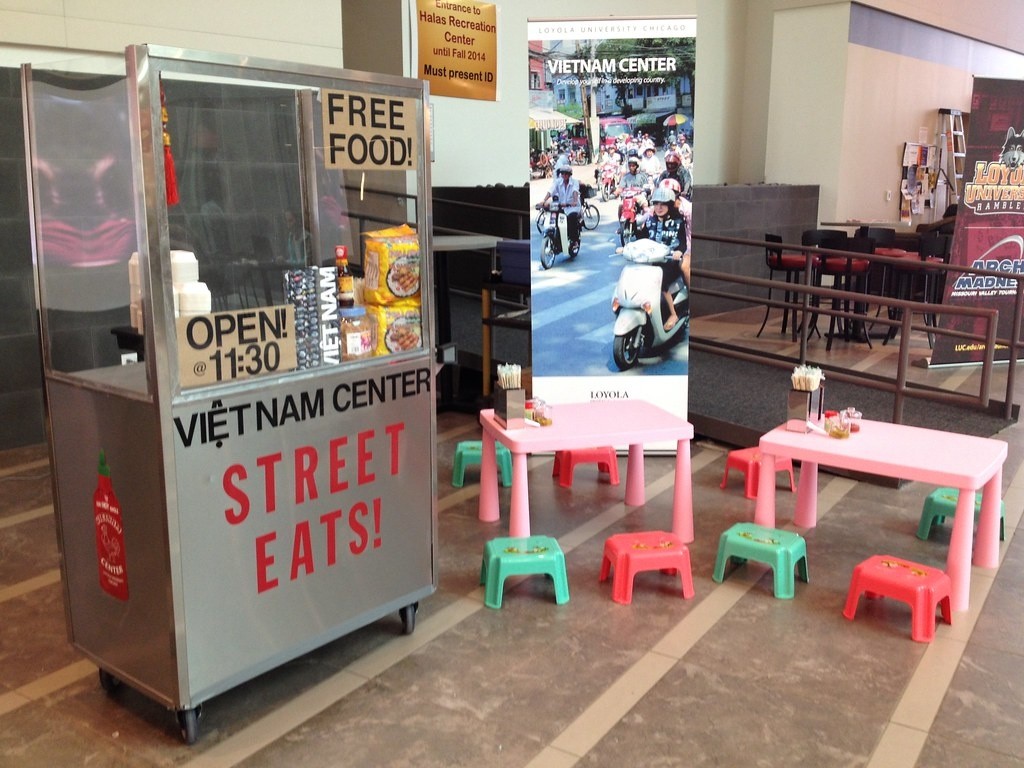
[630,130,649,145]
[650,187,675,203]
[658,178,681,195]
[665,153,682,172]
[608,144,616,150]
[670,141,677,147]
[678,135,686,142]
[628,156,640,170]
[560,164,572,176]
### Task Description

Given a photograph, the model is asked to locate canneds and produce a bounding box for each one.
[337,303,372,362]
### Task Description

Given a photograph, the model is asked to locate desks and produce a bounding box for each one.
[432,237,500,416]
[754,413,1009,609]
[479,398,695,545]
[820,219,910,341]
[230,264,292,308]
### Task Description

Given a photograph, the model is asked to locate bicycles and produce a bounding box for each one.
[535,184,600,234]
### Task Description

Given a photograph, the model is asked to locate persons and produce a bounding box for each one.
[594,130,662,233]
[656,133,692,207]
[615,178,692,331]
[530,135,594,255]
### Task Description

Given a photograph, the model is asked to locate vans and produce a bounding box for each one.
[569,117,634,151]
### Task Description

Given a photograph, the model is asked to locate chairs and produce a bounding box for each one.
[498,240,531,320]
[201,254,266,311]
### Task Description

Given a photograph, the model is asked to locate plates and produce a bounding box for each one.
[386,256,420,298]
[384,316,423,354]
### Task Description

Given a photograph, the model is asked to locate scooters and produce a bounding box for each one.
[610,185,649,248]
[608,237,690,372]
[535,140,689,202]
[536,194,583,269]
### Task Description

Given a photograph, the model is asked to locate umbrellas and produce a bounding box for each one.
[663,113,689,127]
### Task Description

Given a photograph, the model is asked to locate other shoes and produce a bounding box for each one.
[664,316,679,331]
[617,226,626,234]
[572,240,578,253]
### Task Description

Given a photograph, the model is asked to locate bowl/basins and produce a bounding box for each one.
[129,249,211,334]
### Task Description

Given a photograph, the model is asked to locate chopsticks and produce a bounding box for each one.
[792,364,823,391]
[498,362,521,389]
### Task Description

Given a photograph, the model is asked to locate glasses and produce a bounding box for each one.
[652,201,669,206]
[628,164,636,167]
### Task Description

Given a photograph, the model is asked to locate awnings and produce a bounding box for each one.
[529,109,583,129]
[627,111,675,124]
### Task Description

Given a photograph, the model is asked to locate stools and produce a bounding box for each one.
[917,488,1005,542]
[451,441,512,490]
[843,555,953,642]
[711,522,810,600]
[552,446,619,488]
[758,226,948,350]
[718,446,796,499]
[478,535,570,609]
[599,530,695,604]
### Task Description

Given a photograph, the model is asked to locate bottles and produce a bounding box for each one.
[334,245,355,308]
[526,396,553,426]
[337,306,372,362]
[824,407,862,437]
